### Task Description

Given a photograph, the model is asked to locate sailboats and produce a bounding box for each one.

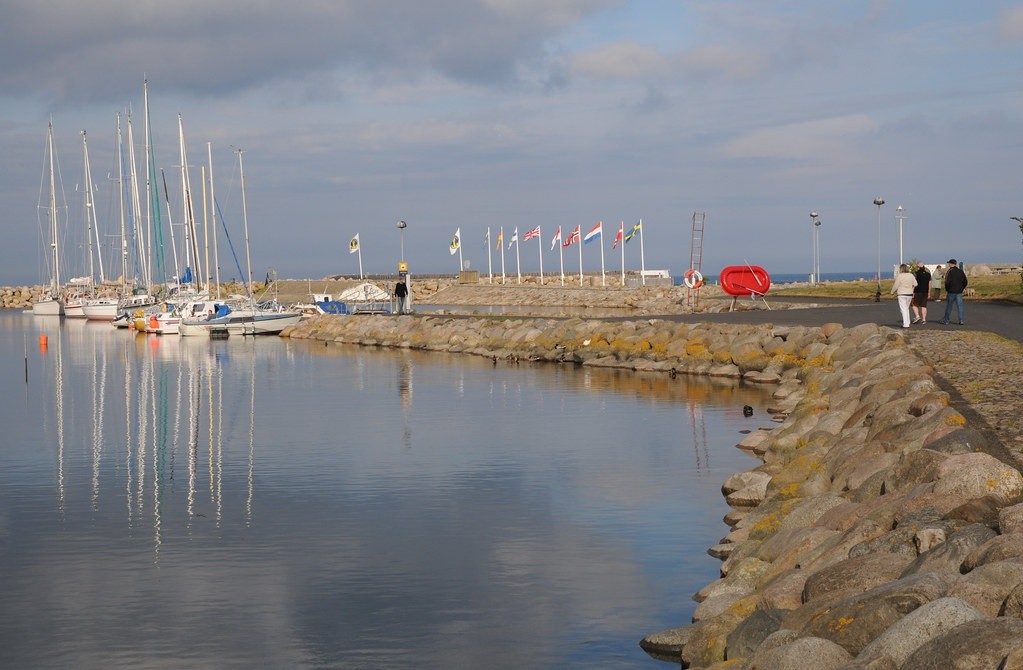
[25,311,282,553]
[31,68,303,337]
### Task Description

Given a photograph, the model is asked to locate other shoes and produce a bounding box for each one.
[938,318,951,324]
[912,316,921,324]
[921,320,926,324]
[934,299,941,302]
[959,320,964,325]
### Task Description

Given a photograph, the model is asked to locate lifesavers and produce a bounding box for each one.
[162,304,166,312]
[134,309,144,318]
[684,270,703,289]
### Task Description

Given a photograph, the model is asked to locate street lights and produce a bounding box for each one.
[894,206,902,264]
[814,221,821,284]
[395,220,407,262]
[873,196,885,287]
[809,210,819,286]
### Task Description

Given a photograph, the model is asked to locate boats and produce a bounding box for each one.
[719,267,770,295]
[312,282,400,317]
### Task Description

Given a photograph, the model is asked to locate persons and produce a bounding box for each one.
[890,264,918,328]
[395,278,408,315]
[204,310,216,322]
[931,265,945,302]
[911,262,931,325]
[940,259,968,325]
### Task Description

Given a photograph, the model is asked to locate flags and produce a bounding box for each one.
[483,219,643,251]
[349,233,360,255]
[449,227,461,255]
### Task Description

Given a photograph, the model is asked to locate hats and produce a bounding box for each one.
[917,262,925,268]
[947,259,957,264]
